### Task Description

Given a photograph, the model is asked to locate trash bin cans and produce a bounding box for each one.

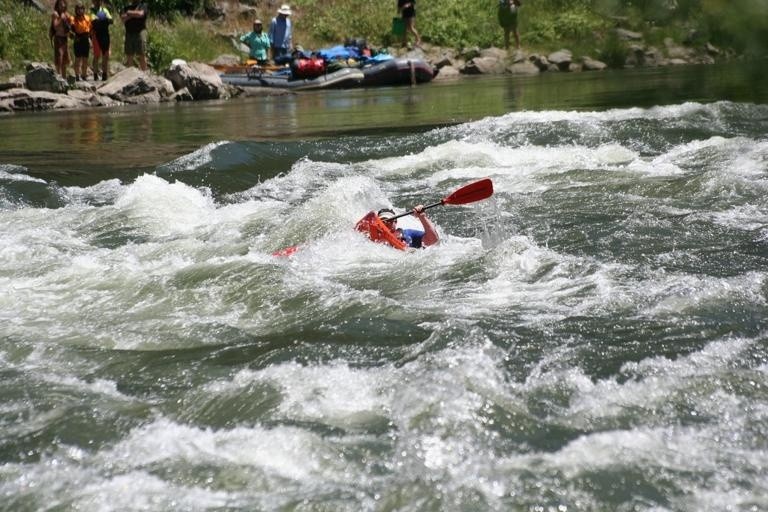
[392,18,406,36]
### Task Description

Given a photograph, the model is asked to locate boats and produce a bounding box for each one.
[208,38,444,95]
[351,208,417,253]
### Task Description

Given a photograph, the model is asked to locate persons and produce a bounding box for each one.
[118,0,147,70]
[397,0,420,47]
[269,4,293,63]
[240,20,271,66]
[499,0,522,50]
[70,4,92,82]
[87,0,114,81]
[377,204,439,249]
[49,0,72,79]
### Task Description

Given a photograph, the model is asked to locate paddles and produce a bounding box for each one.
[209,63,289,73]
[272,179,493,256]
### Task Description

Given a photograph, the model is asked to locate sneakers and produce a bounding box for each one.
[93,72,98,79]
[75,73,79,81]
[101,72,108,80]
[81,74,87,79]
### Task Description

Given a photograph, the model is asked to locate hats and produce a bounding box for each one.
[253,19,263,25]
[278,4,291,16]
[378,212,399,223]
